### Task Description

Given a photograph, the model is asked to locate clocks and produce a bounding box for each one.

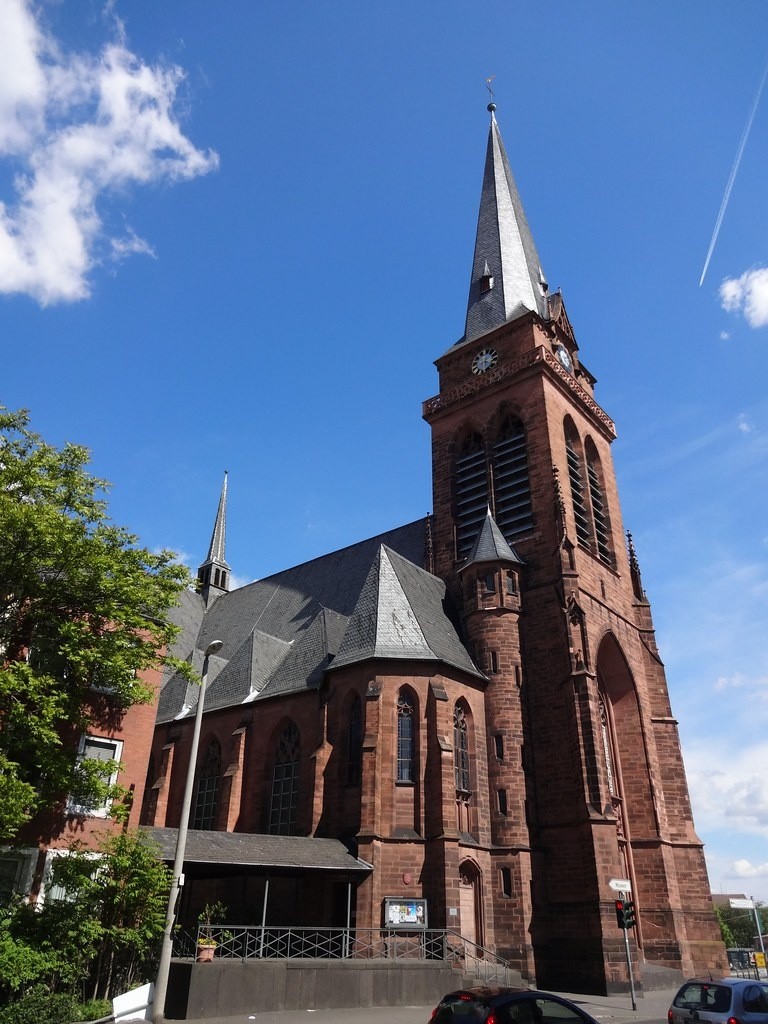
[557,345,572,374]
[471,348,498,375]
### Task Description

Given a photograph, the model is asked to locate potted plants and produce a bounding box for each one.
[195,897,234,964]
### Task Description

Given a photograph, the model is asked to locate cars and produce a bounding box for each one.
[428,986,600,1024]
[667,977,768,1024]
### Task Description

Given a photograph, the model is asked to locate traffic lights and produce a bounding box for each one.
[615,899,637,930]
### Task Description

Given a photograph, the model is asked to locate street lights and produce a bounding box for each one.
[151,637,225,1019]
